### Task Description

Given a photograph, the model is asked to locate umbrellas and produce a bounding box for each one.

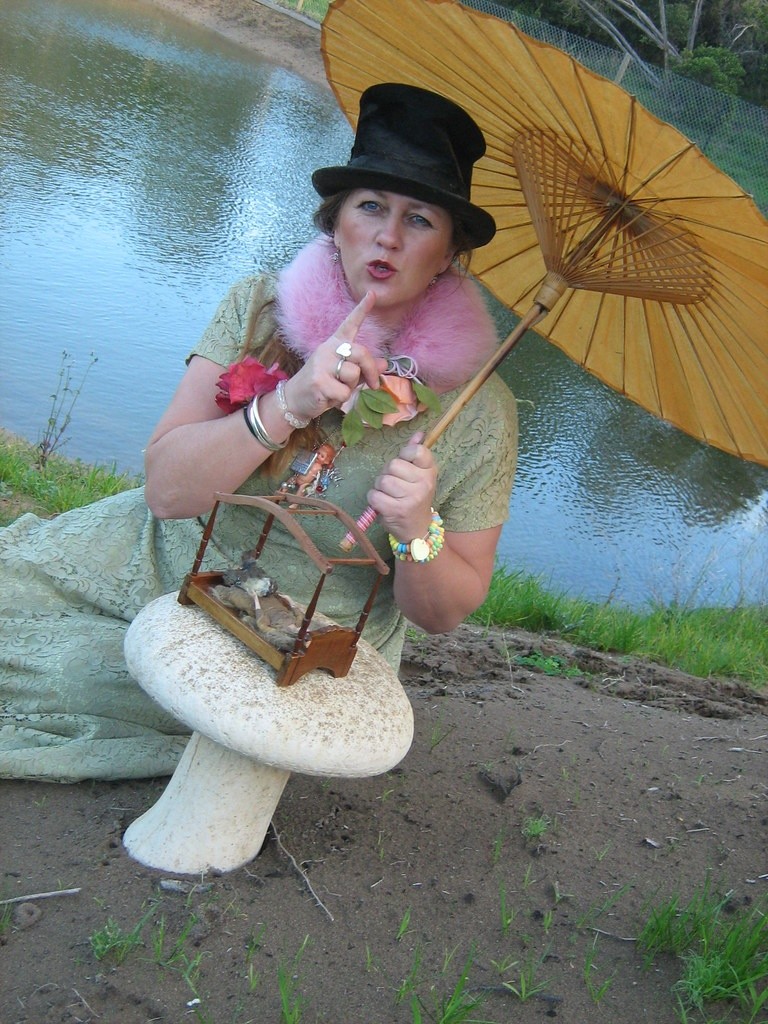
[320,1,768,550]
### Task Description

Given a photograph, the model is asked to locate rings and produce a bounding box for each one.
[335,358,346,380]
[335,343,351,358]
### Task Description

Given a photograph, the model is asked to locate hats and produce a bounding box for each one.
[311,84,496,251]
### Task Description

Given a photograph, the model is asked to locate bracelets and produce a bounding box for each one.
[253,394,290,449]
[247,399,279,451]
[243,405,259,440]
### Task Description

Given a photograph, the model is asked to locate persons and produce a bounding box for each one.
[0,81,520,788]
[273,444,335,509]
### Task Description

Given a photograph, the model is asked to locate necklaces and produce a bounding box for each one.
[273,414,354,509]
[275,379,310,429]
